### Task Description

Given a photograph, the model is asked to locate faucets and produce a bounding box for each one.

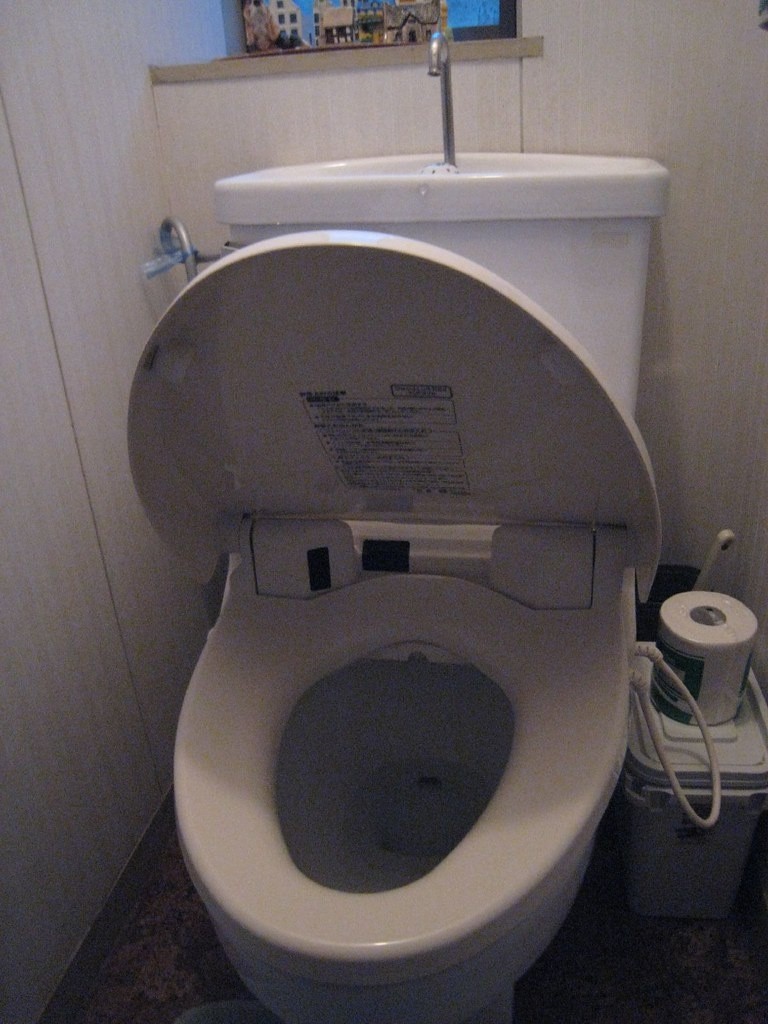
[422,29,459,167]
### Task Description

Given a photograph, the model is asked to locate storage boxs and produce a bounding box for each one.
[619,642,768,918]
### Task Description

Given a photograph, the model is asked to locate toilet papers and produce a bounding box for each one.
[648,588,759,727]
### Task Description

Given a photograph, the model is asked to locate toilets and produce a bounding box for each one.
[124,148,668,1024]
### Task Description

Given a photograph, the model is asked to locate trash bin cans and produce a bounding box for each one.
[623,634,767,924]
